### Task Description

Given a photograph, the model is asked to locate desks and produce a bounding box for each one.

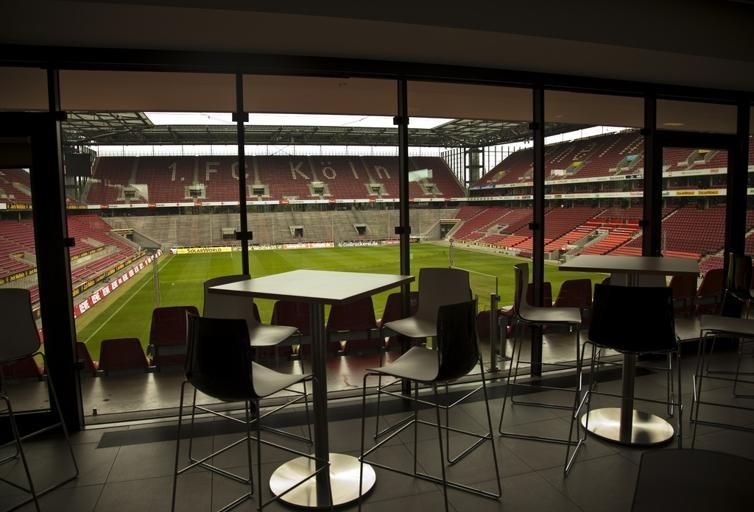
[559,254,698,449]
[206,268,415,512]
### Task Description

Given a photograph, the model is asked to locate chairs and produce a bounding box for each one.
[374,267,490,463]
[376,292,418,349]
[271,301,323,360]
[670,271,700,317]
[690,288,754,397]
[149,306,200,372]
[97,338,154,374]
[77,341,96,375]
[696,269,724,314]
[358,298,502,512]
[172,313,313,511]
[499,263,583,446]
[189,274,315,481]
[591,270,673,416]
[0,289,79,499]
[553,278,590,329]
[501,282,552,338]
[325,296,380,355]
[564,283,681,478]
